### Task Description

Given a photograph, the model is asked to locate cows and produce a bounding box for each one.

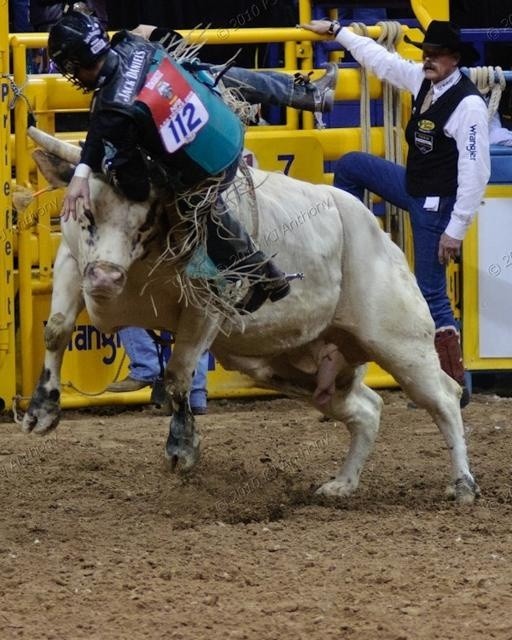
[20,125,481,506]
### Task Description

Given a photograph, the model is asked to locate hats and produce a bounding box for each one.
[401,19,481,67]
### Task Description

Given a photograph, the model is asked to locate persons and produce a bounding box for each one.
[294,14,493,412]
[103,326,212,420]
[45,6,341,315]
[454,41,511,147]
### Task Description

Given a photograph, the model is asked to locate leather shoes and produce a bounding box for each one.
[151,393,207,418]
[105,362,169,393]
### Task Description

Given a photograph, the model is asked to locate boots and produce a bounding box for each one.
[290,61,341,113]
[406,323,471,409]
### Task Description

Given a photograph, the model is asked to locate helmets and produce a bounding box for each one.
[47,10,117,87]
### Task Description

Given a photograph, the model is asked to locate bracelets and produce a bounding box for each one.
[71,163,92,180]
[327,19,339,35]
